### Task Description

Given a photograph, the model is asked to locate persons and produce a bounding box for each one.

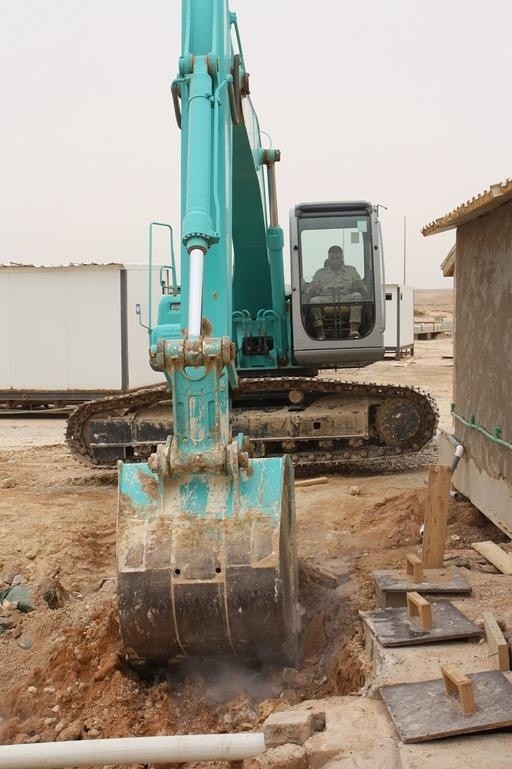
[308,245,366,340]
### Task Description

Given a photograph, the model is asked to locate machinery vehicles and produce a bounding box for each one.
[64,0,441,674]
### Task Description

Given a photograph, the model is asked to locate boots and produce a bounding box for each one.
[314,325,326,339]
[349,321,360,337]
